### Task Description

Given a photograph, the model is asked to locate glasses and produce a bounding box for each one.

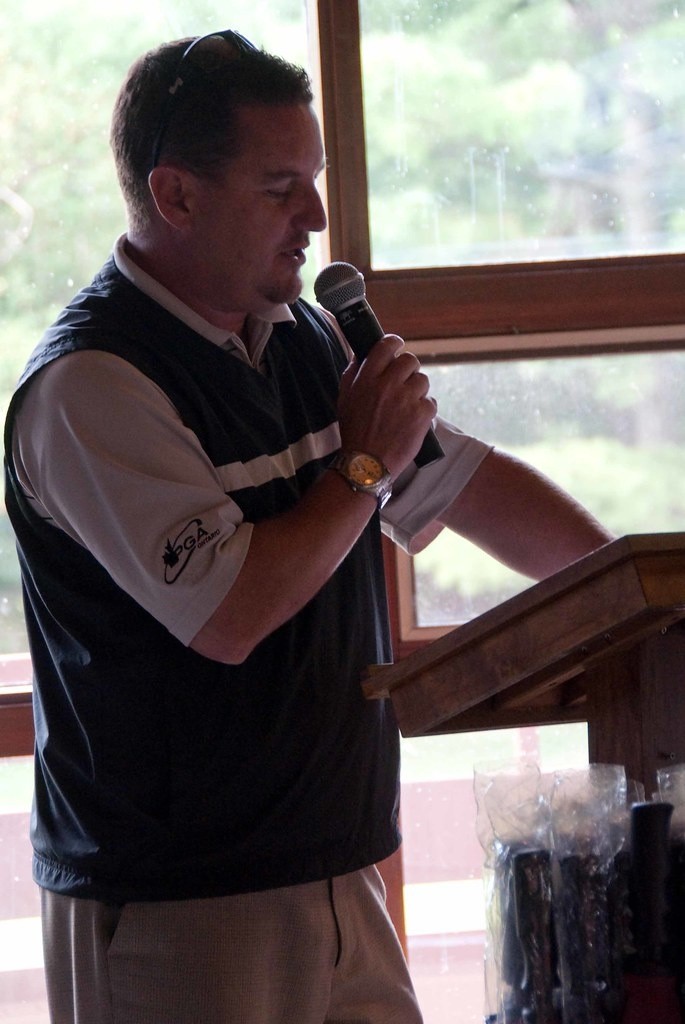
[153,29,259,168]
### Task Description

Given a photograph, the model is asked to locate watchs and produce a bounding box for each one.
[327,447,393,510]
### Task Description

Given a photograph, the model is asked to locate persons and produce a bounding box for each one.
[3,28,616,1024]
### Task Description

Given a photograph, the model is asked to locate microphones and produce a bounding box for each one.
[314,262,446,470]
